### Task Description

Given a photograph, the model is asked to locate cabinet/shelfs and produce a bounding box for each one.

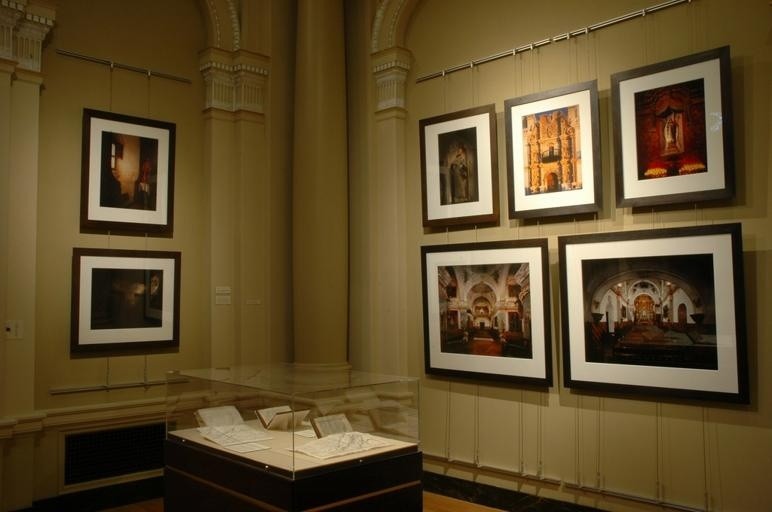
[163,365,424,508]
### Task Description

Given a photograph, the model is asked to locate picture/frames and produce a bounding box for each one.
[609,44,736,208]
[69,245,182,354]
[503,78,602,220]
[80,106,176,239]
[419,237,553,388]
[418,103,500,228]
[557,221,750,410]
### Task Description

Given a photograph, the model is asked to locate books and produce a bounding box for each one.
[195,405,398,460]
